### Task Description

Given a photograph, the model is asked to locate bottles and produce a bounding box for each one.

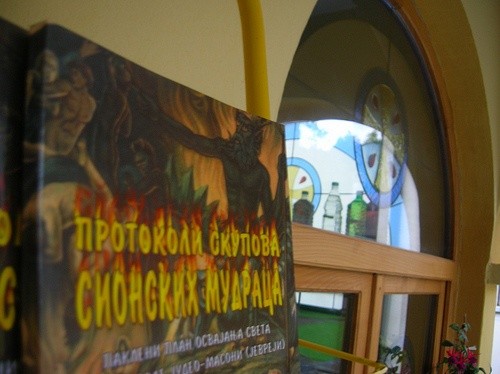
[346,190,368,236]
[292,191,315,224]
[322,183,343,232]
